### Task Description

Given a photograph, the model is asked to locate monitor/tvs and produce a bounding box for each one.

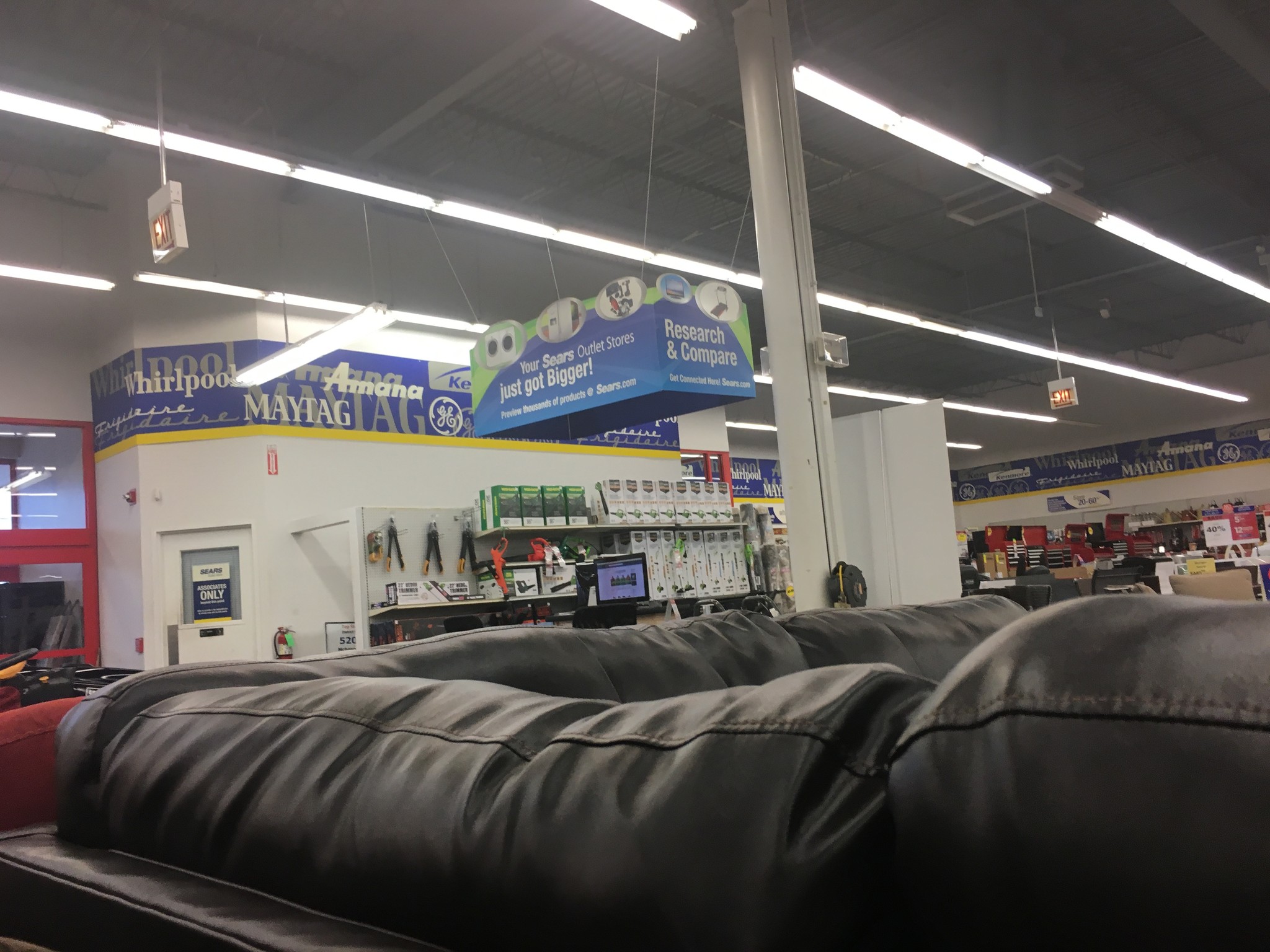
[594,552,650,605]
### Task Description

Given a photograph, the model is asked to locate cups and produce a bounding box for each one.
[1189,543,1198,550]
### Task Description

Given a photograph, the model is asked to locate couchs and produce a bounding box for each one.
[0,594,1270,952]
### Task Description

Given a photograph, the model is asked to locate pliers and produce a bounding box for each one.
[386,518,406,571]
[424,520,444,575]
[457,521,479,574]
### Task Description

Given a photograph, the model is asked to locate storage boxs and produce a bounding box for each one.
[474,486,589,532]
[600,529,750,600]
[478,564,577,600]
[385,581,470,606]
[590,480,733,524]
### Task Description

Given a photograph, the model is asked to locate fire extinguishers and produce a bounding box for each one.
[271,627,297,659]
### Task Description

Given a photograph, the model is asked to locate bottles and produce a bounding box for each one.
[1130,512,1156,521]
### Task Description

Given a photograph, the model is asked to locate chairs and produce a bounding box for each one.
[1168,569,1257,600]
[959,554,1161,610]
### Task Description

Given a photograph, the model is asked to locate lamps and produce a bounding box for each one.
[793,60,1270,304]
[813,331,849,368]
[588,0,699,42]
[1,468,52,495]
[760,346,771,378]
[229,201,396,388]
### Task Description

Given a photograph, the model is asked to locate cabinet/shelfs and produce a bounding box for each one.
[369,522,747,618]
